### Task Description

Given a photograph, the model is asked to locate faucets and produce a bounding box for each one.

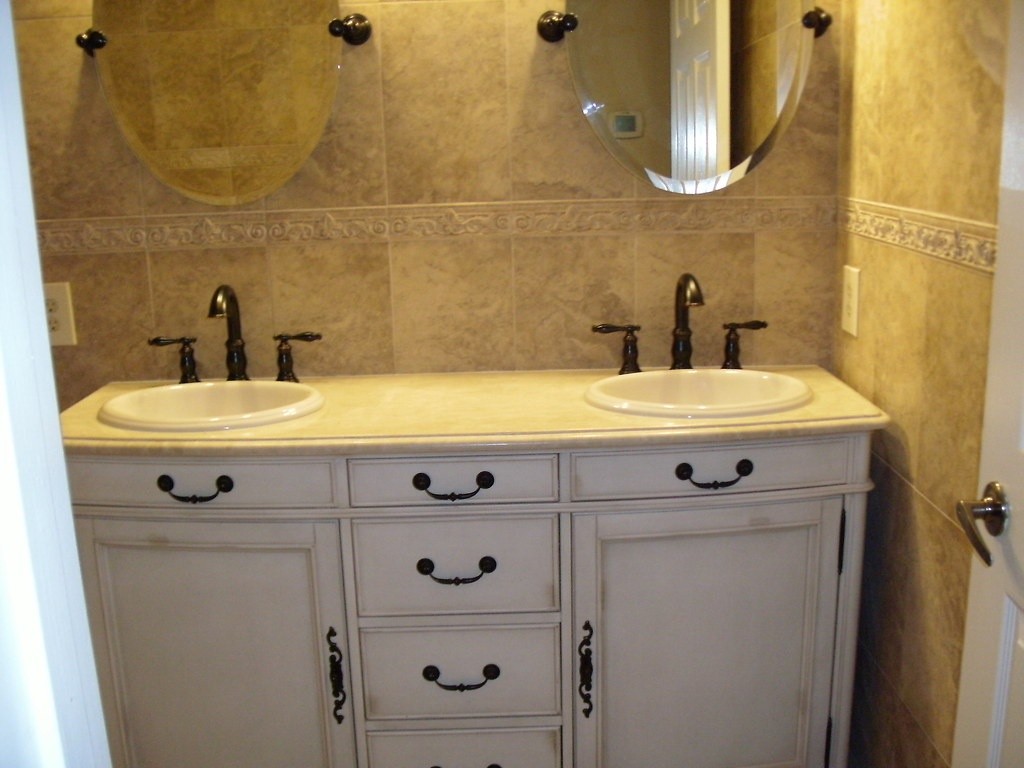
[206,285,248,381]
[671,272,706,370]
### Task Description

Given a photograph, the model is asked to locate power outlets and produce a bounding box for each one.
[841,265,860,336]
[42,281,78,348]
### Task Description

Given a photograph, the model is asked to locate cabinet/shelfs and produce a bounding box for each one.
[62,428,879,768]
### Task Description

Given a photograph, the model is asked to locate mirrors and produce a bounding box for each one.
[92,0,340,207]
[564,0,815,194]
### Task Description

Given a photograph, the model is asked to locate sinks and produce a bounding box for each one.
[584,368,813,420]
[98,380,321,430]
